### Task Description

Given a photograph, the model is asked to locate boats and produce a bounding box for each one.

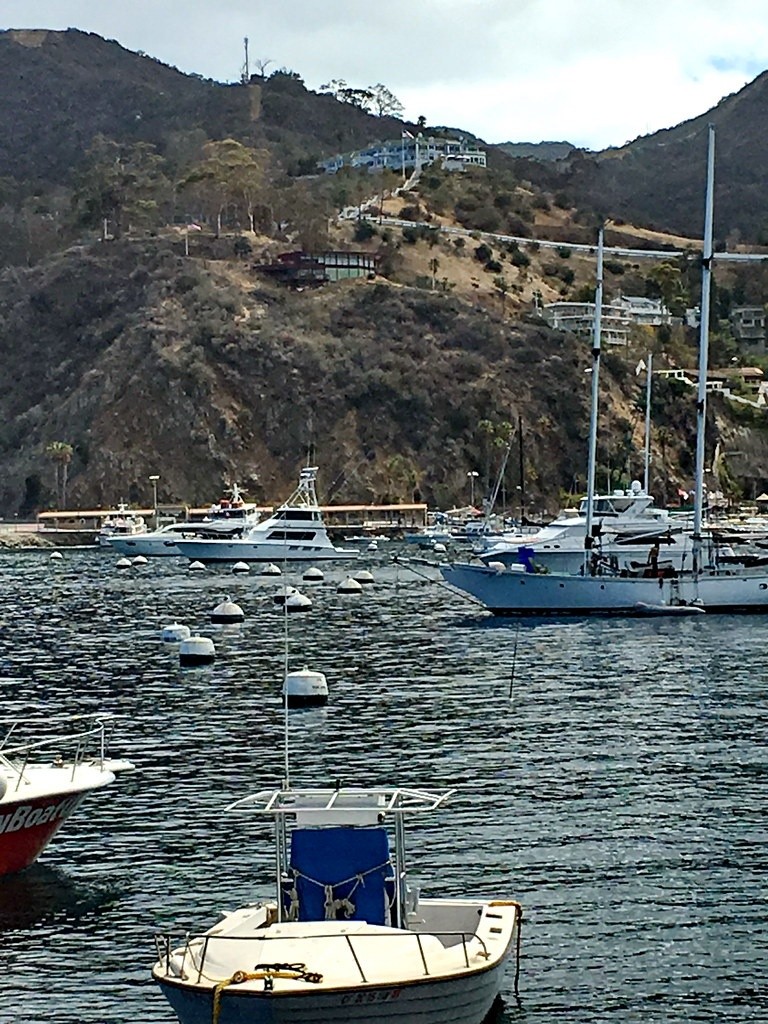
[0,718,136,876]
[101,461,360,568]
[150,511,518,1024]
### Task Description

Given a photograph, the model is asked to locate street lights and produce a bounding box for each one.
[467,471,479,505]
[149,475,159,508]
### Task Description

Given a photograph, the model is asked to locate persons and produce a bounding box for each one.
[588,548,603,577]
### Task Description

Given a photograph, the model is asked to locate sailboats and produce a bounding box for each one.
[478,353,734,573]
[439,120,768,620]
[404,410,540,550]
